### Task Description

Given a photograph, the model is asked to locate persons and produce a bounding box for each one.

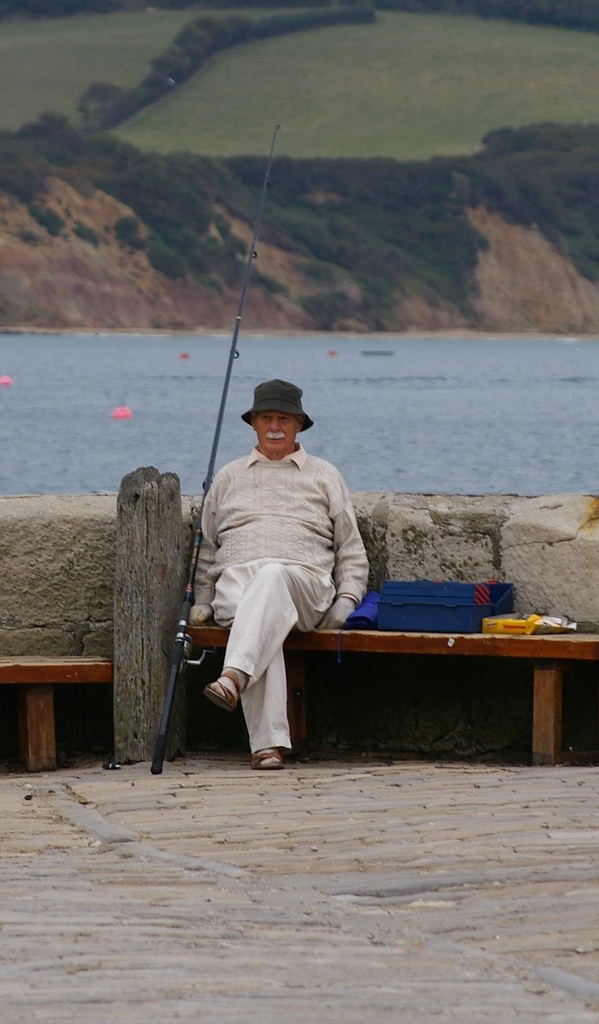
[190,379,369,769]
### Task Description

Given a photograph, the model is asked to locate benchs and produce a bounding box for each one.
[186,625,599,766]
[0,655,113,772]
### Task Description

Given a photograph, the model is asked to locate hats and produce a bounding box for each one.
[241,379,313,433]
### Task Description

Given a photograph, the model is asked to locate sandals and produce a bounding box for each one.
[203,673,239,711]
[250,750,283,769]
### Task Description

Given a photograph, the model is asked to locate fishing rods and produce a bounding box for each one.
[147,115,291,776]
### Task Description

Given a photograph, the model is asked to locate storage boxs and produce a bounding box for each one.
[376,580,578,636]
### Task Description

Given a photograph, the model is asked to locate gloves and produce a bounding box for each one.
[188,604,212,626]
[317,597,355,628]
